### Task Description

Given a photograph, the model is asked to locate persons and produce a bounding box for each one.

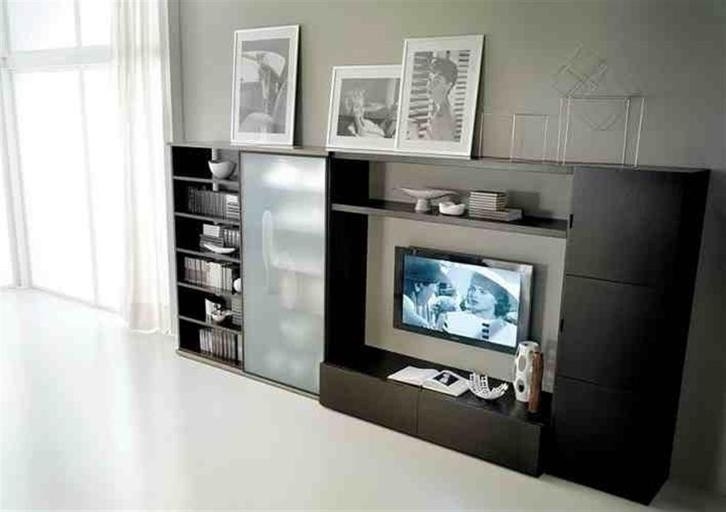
[239,51,288,133]
[403,254,443,327]
[340,90,384,139]
[423,58,459,139]
[437,266,521,347]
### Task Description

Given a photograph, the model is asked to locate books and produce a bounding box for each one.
[182,187,241,364]
[387,366,472,397]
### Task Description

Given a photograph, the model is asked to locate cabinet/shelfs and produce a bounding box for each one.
[319,142,574,479]
[169,144,244,371]
[545,152,711,505]
[239,151,369,395]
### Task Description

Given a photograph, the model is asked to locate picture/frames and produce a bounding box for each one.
[324,65,402,151]
[230,24,301,147]
[394,32,485,158]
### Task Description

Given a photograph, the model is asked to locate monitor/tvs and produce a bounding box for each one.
[391,245,536,359]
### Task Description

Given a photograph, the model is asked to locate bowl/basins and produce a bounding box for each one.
[208,159,235,179]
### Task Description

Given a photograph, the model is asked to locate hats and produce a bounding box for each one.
[461,266,519,306]
[405,258,441,282]
